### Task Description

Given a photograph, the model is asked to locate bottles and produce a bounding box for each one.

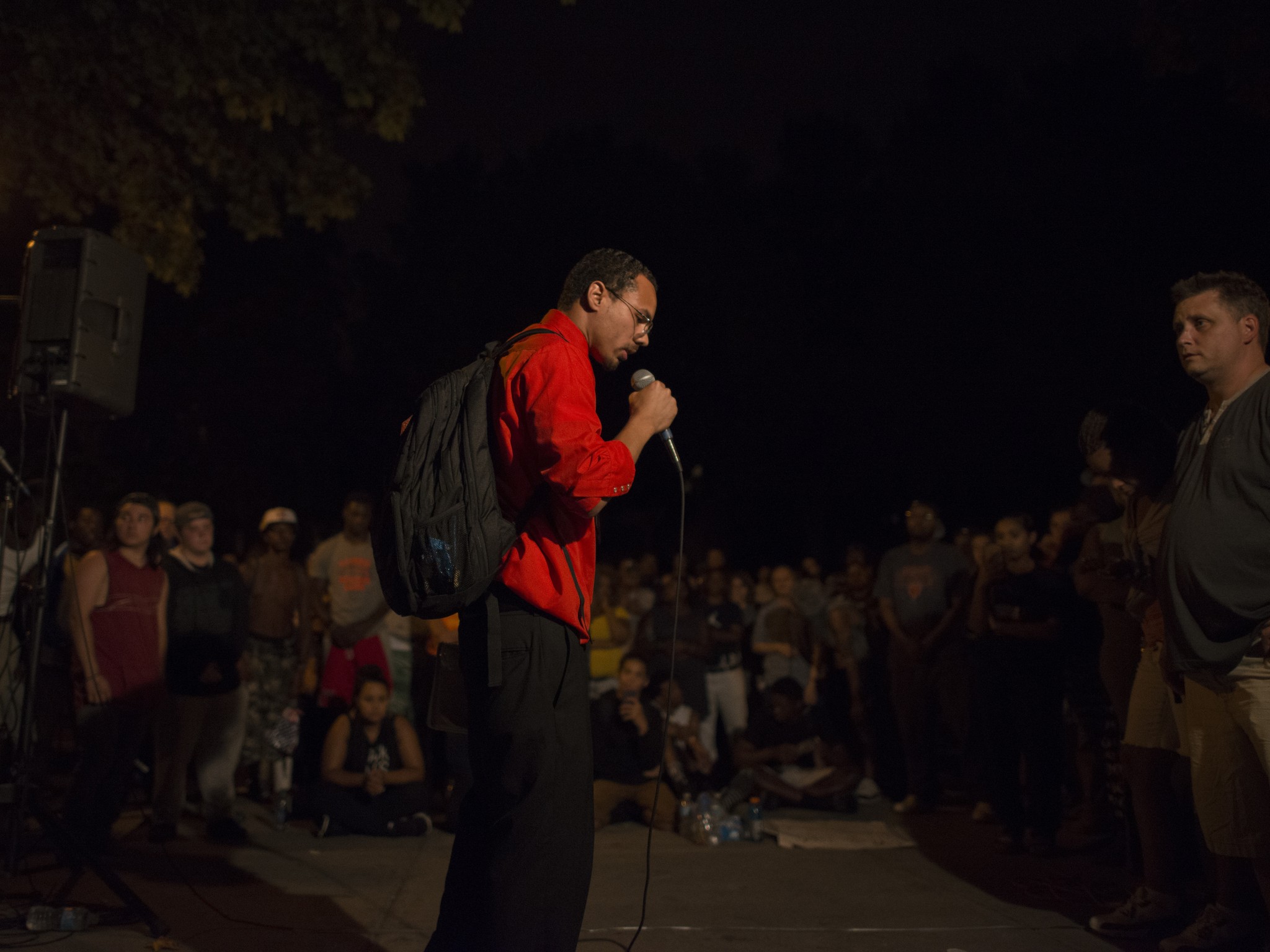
[676,791,762,845]
[24,905,101,931]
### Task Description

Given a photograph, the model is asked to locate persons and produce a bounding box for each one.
[0,421,1270,869]
[419,250,679,952]
[1166,271,1270,952]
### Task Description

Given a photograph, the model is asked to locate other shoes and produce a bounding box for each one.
[761,773,1163,880]
[148,818,177,844]
[207,816,252,846]
[387,812,433,836]
[317,813,356,837]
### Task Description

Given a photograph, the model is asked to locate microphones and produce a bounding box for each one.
[631,368,685,473]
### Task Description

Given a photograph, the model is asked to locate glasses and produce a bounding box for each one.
[604,286,654,333]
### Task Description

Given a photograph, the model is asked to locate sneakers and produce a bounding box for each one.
[1089,886,1178,939]
[1160,901,1244,952]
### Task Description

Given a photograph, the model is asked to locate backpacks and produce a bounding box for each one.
[368,328,569,619]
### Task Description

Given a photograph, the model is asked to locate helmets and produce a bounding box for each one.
[259,507,299,534]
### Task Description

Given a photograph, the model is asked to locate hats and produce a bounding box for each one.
[176,500,213,531]
[1079,447,1146,486]
[115,492,160,524]
